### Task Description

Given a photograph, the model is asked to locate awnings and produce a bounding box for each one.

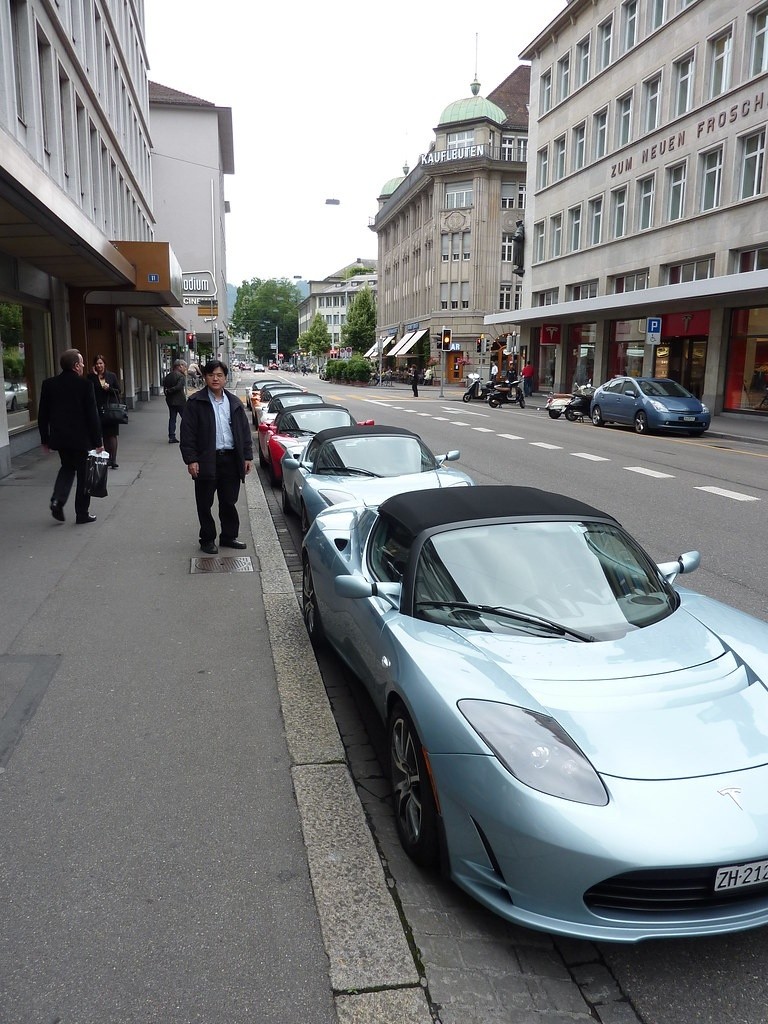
[363,337,386,357]
[395,328,429,356]
[370,335,395,357]
[385,329,416,357]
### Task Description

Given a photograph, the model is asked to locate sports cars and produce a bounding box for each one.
[257,402,377,482]
[301,483,768,942]
[282,423,474,537]
[3,380,29,412]
[244,380,325,431]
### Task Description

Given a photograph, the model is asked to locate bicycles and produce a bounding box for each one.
[186,374,205,391]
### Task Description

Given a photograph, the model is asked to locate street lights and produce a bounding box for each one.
[262,326,279,366]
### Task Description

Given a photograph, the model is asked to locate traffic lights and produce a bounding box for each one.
[483,337,492,354]
[476,338,482,353]
[441,329,451,352]
[435,332,442,351]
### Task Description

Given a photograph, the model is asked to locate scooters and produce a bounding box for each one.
[544,378,597,420]
[463,376,496,402]
[484,377,526,410]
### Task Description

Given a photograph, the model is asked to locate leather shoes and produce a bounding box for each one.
[76,514,97,524]
[219,538,247,549]
[50,500,66,522]
[200,542,219,554]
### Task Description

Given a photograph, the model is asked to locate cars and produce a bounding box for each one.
[269,363,278,370]
[281,362,298,373]
[591,376,712,432]
[233,360,252,371]
[254,364,266,372]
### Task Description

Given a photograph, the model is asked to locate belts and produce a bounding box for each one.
[217,449,237,455]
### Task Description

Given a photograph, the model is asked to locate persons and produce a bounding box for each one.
[188,363,202,391]
[179,360,254,555]
[489,361,498,382]
[423,368,432,386]
[163,359,187,443]
[90,354,123,469]
[522,360,536,398]
[38,349,105,524]
[301,363,309,376]
[239,362,244,370]
[370,362,411,385]
[505,363,517,383]
[408,364,419,397]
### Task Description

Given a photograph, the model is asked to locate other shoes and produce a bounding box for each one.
[169,438,180,443]
[111,464,119,468]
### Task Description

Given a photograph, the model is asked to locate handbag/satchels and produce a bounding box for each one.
[98,388,129,425]
[86,449,108,498]
